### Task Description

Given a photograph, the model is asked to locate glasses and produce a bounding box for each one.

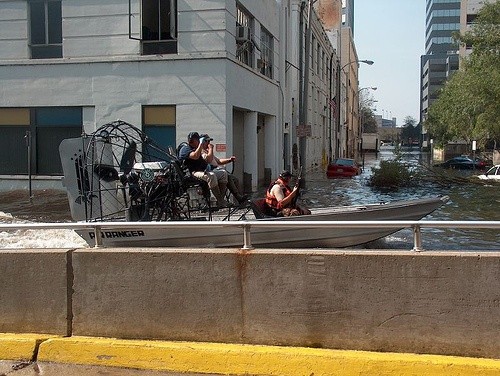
[205,140,210,142]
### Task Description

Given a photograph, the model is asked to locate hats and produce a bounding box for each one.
[200,134,213,141]
[189,133,202,139]
[280,171,295,179]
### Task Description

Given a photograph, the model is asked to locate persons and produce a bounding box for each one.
[266,170,311,217]
[177,132,237,208]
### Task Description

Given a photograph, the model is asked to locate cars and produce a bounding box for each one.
[325,157,363,178]
[477,164,500,181]
[433,157,493,170]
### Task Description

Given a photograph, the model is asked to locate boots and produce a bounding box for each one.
[216,182,226,205]
[209,185,235,209]
[226,175,248,205]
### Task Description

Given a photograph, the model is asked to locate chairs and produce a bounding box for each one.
[167,142,277,221]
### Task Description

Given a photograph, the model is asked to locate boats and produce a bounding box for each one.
[72,194,454,249]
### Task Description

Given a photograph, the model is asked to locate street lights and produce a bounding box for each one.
[336,60,375,162]
[352,85,377,154]
[359,97,379,152]
